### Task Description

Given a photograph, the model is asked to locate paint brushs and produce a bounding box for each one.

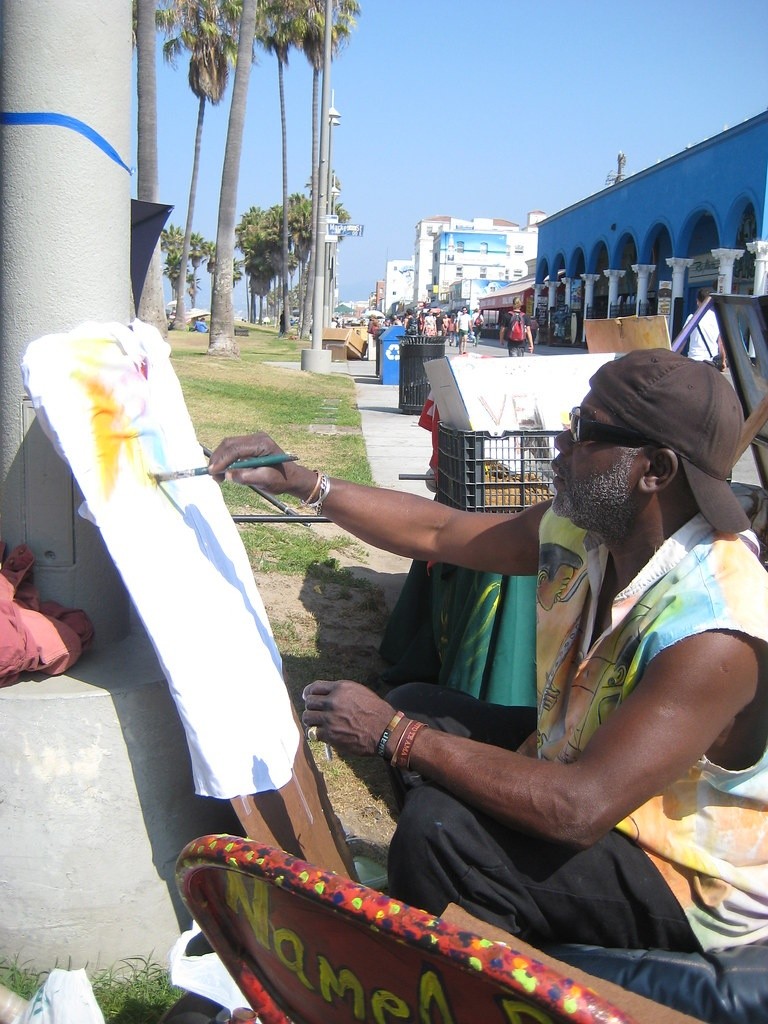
[149,453,303,491]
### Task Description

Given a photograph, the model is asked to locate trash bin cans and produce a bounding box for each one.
[377,323,407,387]
[396,335,450,415]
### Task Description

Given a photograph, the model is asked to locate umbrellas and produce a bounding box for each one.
[185,307,211,318]
[334,305,355,315]
[167,300,186,309]
[363,310,386,319]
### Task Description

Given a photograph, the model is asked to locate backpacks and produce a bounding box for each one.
[508,311,526,342]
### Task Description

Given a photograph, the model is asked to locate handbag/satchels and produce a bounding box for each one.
[712,353,728,371]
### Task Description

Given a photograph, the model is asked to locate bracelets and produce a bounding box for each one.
[391,720,429,774]
[377,711,404,758]
[301,470,330,516]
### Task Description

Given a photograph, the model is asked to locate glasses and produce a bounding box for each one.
[568,405,660,447]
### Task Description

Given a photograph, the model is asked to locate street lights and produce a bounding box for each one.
[326,89,340,330]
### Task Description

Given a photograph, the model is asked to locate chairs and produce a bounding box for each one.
[172,833,635,1024]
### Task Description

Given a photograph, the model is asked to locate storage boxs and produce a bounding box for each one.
[435,420,566,514]
[322,328,368,360]
[326,343,347,360]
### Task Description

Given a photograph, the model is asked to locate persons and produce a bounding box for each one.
[194,317,209,333]
[674,288,727,373]
[280,309,341,334]
[206,348,768,955]
[368,307,484,354]
[500,298,539,357]
[169,308,177,319]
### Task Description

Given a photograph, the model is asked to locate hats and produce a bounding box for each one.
[588,347,751,533]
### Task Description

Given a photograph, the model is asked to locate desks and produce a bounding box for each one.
[378,561,537,707]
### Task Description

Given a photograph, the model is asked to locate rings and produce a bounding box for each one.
[307,726,318,743]
